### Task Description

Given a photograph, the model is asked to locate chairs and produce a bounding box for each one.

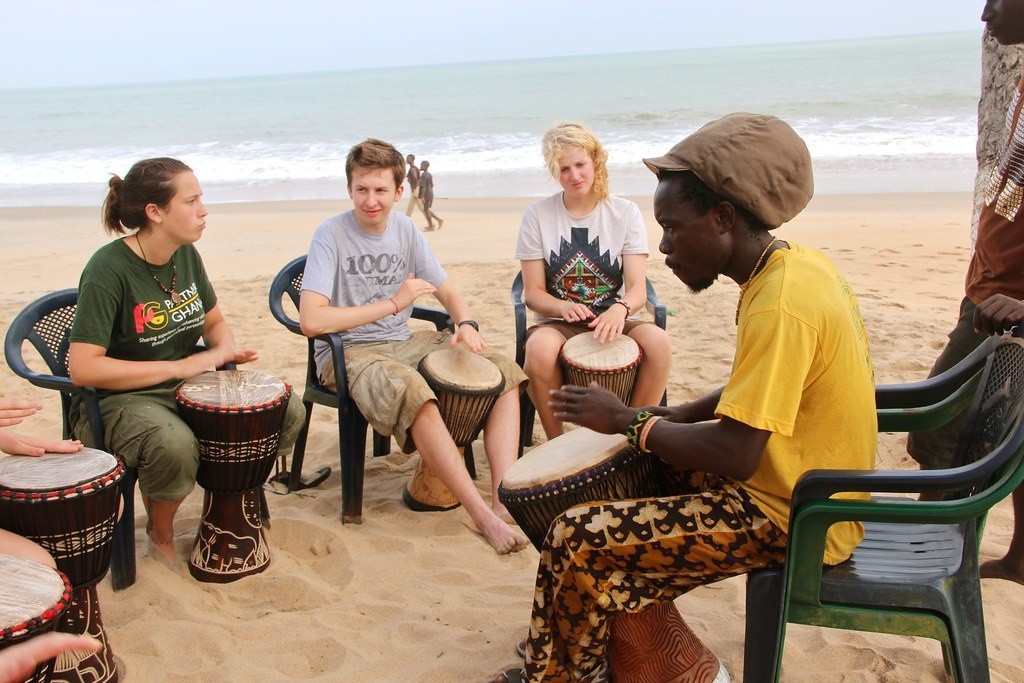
[511,267,670,455]
[739,335,1024,683]
[268,255,475,526]
[3,289,139,592]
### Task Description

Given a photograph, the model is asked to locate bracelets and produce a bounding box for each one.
[616,300,631,319]
[389,297,399,316]
[627,410,663,452]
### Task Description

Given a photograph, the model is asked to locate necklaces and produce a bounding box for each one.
[136,231,182,303]
[734,236,776,325]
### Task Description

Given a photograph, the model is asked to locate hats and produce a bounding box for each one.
[642,112,814,230]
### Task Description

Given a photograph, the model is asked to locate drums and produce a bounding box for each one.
[402,348,508,513]
[1,447,126,683]
[559,331,644,407]
[176,370,293,583]
[0,552,76,683]
[497,427,731,682]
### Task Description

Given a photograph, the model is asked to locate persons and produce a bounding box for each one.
[490,112,878,683]
[299,138,526,555]
[515,123,672,440]
[417,161,443,231]
[69,157,306,557]
[906,0,1024,585]
[404,154,424,217]
[0,398,124,570]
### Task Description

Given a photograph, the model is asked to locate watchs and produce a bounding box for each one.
[460,320,479,332]
